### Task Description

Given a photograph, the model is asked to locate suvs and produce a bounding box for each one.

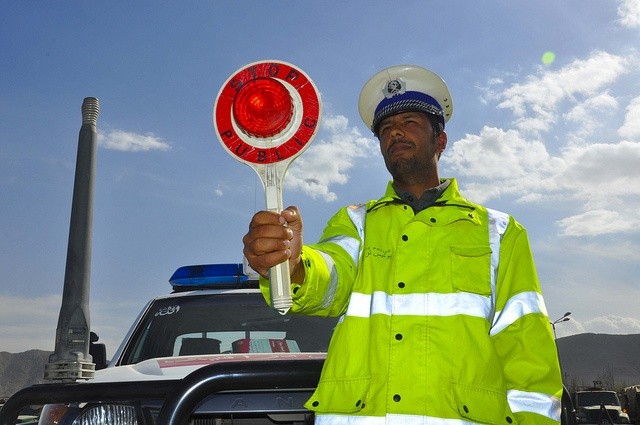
[0,264,347,424]
[573,391,631,424]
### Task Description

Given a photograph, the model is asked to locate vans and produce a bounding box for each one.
[619,385,639,424]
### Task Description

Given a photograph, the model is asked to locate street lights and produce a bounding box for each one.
[549,311,571,385]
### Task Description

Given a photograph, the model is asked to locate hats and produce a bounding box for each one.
[358,65,452,138]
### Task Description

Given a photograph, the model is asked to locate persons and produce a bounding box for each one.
[243,64,564,424]
